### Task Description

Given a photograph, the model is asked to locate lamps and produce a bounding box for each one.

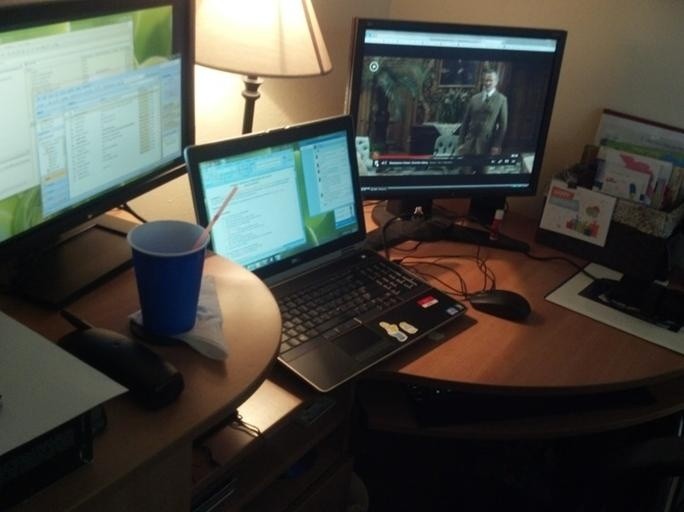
[191,0,335,135]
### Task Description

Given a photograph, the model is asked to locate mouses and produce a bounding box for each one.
[467,286,532,325]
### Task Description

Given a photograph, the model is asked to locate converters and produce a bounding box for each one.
[612,273,684,327]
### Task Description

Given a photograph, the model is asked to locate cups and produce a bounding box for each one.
[126,220,210,335]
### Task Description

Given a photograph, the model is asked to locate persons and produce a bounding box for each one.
[460,68,509,175]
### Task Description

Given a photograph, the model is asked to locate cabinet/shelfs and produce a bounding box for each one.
[0,215,372,512]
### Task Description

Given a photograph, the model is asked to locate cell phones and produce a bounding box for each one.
[57,308,185,414]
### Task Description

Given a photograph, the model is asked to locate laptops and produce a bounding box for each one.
[182,113,468,395]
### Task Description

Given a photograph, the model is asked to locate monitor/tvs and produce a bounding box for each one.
[0,0,196,311]
[342,17,568,255]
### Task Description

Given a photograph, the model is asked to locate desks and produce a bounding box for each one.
[355,200,683,512]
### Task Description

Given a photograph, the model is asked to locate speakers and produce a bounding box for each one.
[469,197,506,225]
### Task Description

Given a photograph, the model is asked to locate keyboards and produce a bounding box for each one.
[402,374,656,427]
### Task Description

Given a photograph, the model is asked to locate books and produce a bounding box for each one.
[596,139,681,211]
